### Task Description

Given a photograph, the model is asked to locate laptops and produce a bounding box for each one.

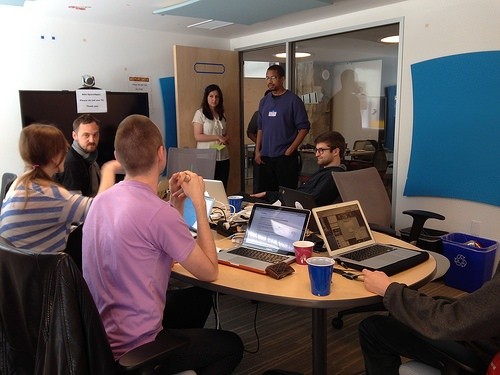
[202,179,232,219]
[182,195,216,238]
[215,203,311,274]
[313,200,421,270]
[278,186,312,211]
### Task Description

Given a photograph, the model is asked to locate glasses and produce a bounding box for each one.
[314,148,329,154]
[266,76,277,80]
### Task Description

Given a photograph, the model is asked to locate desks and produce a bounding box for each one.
[171,202,437,375]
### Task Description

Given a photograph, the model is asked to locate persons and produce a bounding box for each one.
[246,65,311,194]
[358,269,500,375]
[250,131,348,208]
[192,84,230,192]
[0,124,126,252]
[82,114,245,375]
[326,69,362,128]
[51,115,101,198]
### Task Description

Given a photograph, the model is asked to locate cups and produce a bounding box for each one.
[228,195,243,212]
[293,241,315,265]
[306,257,336,296]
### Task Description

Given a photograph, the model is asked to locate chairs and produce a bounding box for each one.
[0,147,500,375]
